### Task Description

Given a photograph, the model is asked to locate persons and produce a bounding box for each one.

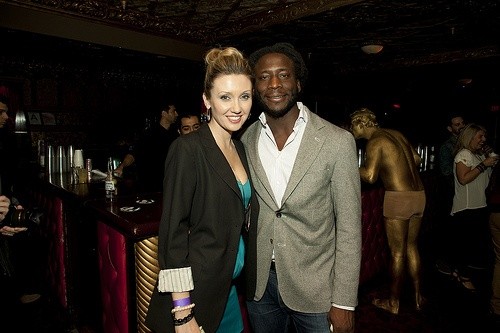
[449,124,500,289]
[346,103,427,314]
[176,110,203,136]
[145,47,259,333]
[114,100,182,205]
[484,160,500,316]
[239,43,361,333]
[0,93,9,130]
[0,171,29,292]
[433,114,467,275]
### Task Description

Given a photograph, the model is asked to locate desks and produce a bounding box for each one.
[29,161,166,333]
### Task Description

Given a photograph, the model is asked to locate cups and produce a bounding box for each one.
[46,142,91,183]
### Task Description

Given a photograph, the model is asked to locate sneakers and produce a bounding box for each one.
[437,265,459,277]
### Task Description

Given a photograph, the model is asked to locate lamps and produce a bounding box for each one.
[357,3,385,55]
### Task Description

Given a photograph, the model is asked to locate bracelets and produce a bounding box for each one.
[171,310,194,326]
[172,297,191,307]
[171,303,195,314]
[477,163,487,172]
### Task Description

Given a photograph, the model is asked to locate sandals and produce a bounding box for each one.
[457,275,479,293]
